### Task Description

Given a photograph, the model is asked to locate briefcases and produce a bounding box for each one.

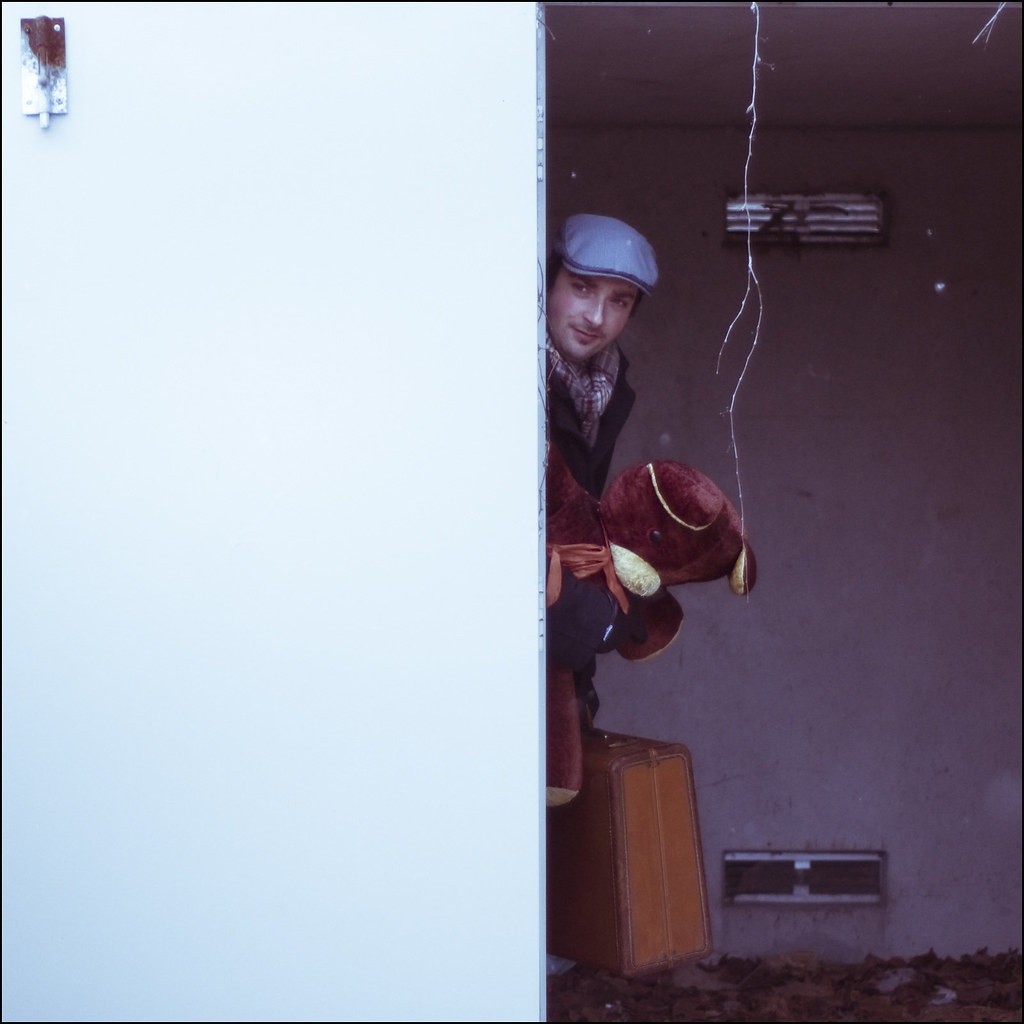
[546,702,713,977]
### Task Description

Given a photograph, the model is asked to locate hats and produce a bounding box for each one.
[551,214,659,299]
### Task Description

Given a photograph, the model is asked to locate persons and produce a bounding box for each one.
[546,211,659,977]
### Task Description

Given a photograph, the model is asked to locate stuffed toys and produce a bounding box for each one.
[546,445,760,805]
